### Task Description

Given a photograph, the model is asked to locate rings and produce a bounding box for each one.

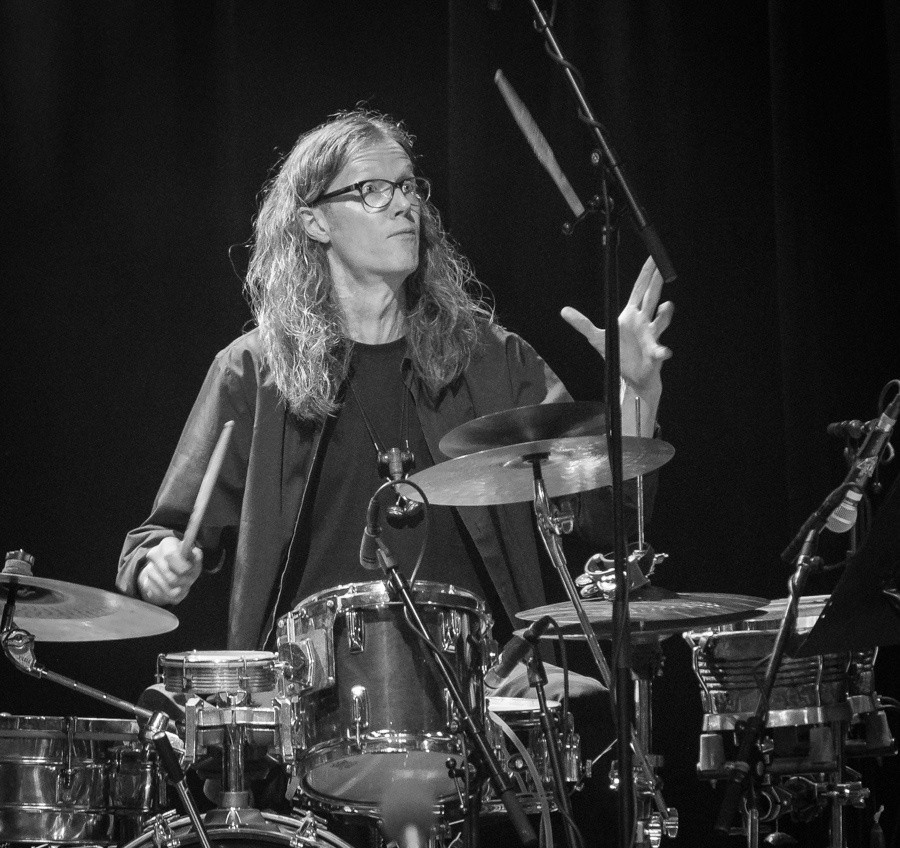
[145,590,153,600]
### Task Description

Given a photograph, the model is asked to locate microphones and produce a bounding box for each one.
[360,497,383,570]
[381,779,437,848]
[825,392,900,533]
[482,616,549,696]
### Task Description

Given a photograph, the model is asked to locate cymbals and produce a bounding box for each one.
[396,399,679,507]
[0,571,181,645]
[513,590,773,640]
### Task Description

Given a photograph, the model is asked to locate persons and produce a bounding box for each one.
[115,115,673,847]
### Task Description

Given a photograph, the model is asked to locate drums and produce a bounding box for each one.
[481,695,574,815]
[683,593,873,734]
[1,712,149,846]
[287,576,487,812]
[127,809,355,847]
[162,648,279,694]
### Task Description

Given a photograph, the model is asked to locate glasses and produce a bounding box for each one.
[308,176,432,208]
